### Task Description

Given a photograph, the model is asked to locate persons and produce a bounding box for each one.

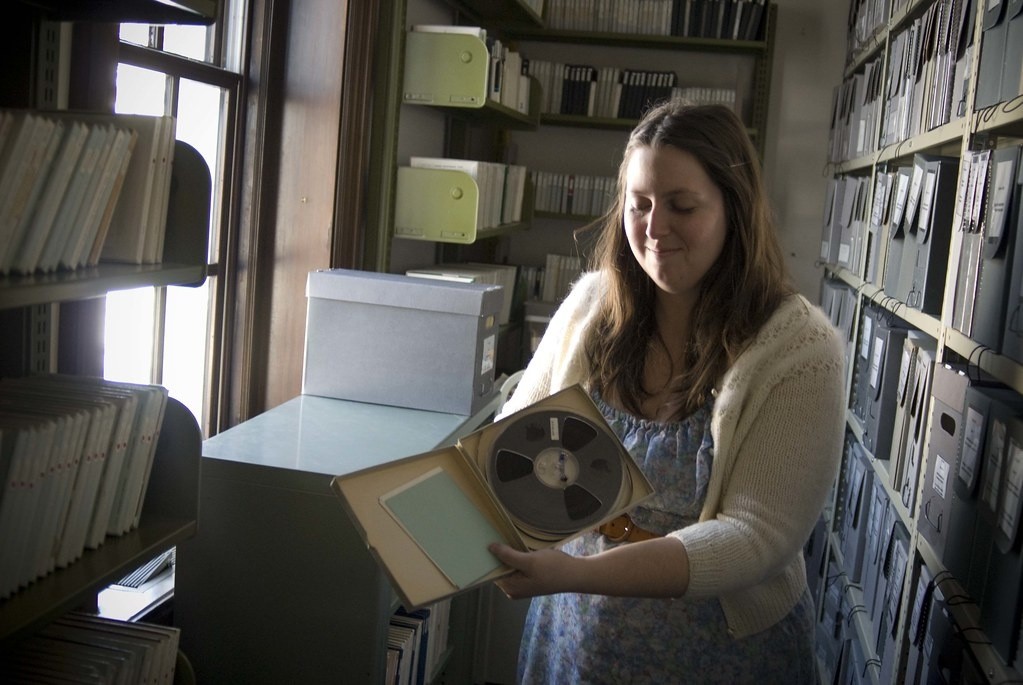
[488,102,847,685]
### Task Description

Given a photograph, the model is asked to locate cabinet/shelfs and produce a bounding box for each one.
[359,0,541,382]
[0,1,225,685]
[512,0,778,368]
[173,393,494,685]
[792,0,1023,685]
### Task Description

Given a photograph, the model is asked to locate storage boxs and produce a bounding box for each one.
[301,267,505,417]
